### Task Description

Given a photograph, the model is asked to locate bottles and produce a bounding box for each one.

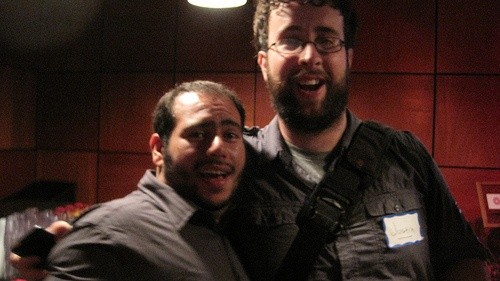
[54,202,86,222]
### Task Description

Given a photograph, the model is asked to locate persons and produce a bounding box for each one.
[43,80,249,281]
[6,0,488,281]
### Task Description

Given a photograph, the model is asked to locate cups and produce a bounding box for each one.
[4,207,58,279]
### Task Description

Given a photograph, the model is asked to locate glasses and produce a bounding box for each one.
[261,37,350,54]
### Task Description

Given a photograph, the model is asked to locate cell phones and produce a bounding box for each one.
[11,225,56,269]
